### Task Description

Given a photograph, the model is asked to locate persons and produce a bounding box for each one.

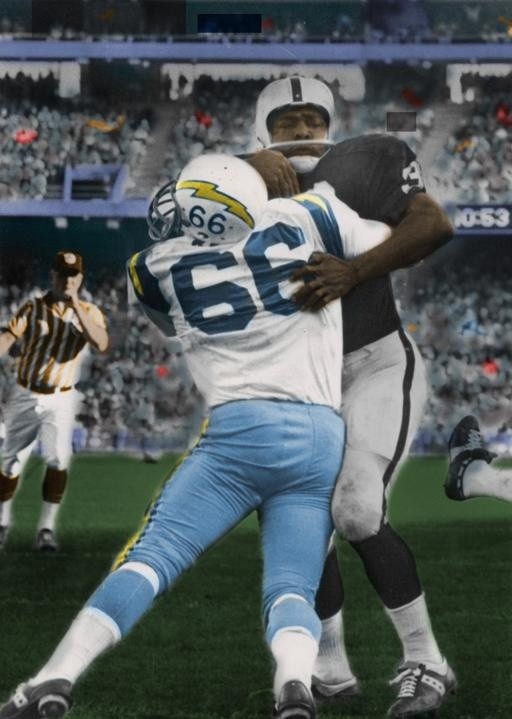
[143,70,462,719]
[1,265,511,464]
[1,0,511,206]
[1,150,400,718]
[0,247,110,552]
[442,414,510,504]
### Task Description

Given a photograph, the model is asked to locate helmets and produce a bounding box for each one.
[169,151,271,243]
[248,73,340,175]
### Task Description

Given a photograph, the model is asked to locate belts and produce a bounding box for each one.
[14,376,77,396]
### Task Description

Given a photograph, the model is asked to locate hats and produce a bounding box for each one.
[51,248,84,273]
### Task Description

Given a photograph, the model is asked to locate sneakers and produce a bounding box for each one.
[31,527,57,552]
[0,677,76,719]
[384,650,462,718]
[0,521,10,553]
[309,674,362,699]
[272,680,317,719]
[441,414,497,502]
